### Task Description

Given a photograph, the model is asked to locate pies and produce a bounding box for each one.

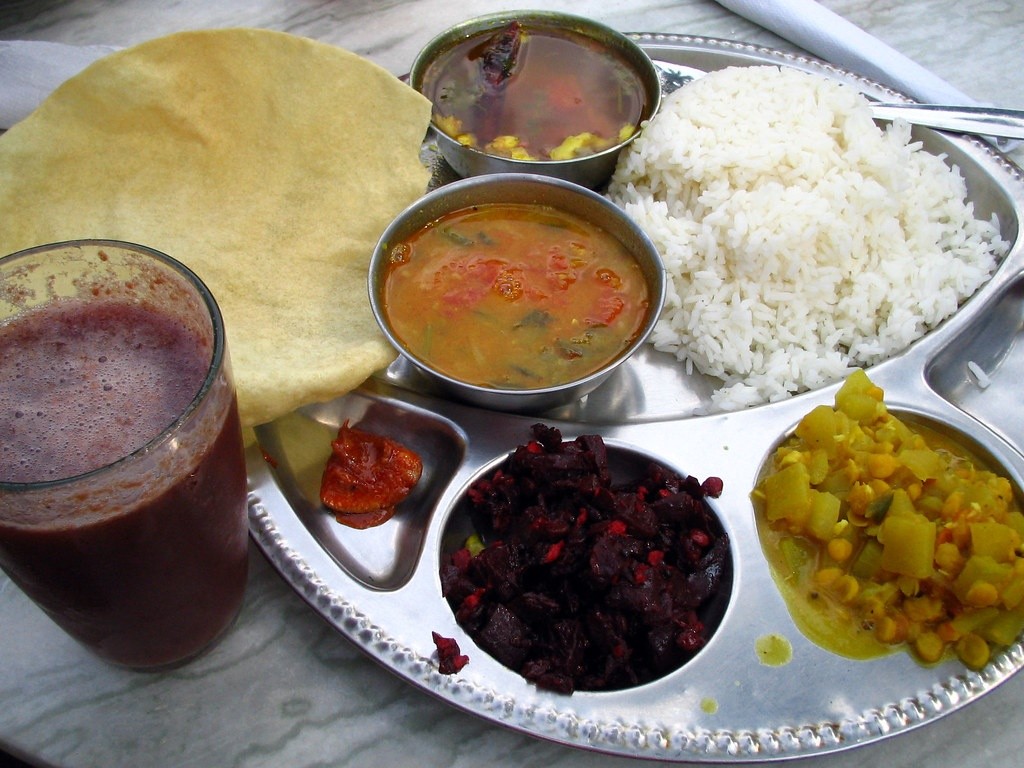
[0,27,436,421]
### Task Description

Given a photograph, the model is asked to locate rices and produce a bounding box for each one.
[599,58,1013,415]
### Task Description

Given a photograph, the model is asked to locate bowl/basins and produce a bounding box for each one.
[368,173,666,412]
[410,9,661,195]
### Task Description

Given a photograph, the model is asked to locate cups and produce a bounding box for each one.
[0,239,252,672]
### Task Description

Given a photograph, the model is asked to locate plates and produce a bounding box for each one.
[249,33,1024,768]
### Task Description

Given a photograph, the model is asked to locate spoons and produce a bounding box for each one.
[650,58,1024,141]
